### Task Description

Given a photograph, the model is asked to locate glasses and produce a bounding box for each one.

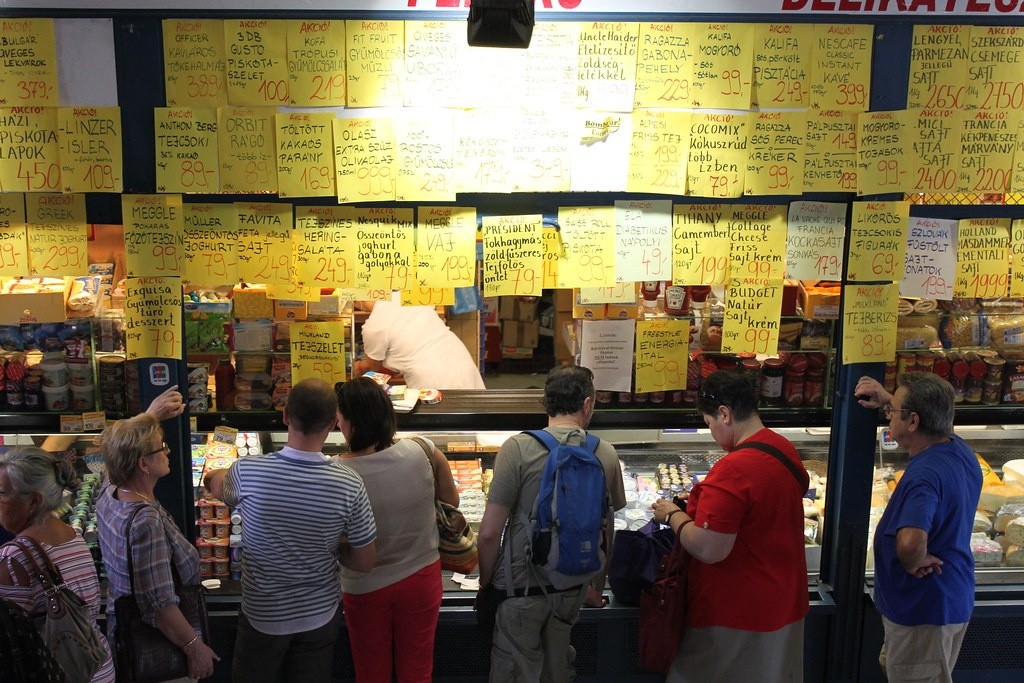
[883,404,911,416]
[334,381,347,402]
[145,440,169,456]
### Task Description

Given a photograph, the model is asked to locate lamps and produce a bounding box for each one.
[467,0,537,49]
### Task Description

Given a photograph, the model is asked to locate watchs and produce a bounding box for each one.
[665,510,684,526]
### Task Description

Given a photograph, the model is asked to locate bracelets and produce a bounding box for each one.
[181,634,199,650]
[359,361,365,375]
[478,578,486,589]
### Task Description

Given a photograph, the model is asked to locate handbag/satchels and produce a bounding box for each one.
[607,517,682,608]
[636,518,694,675]
[115,586,213,683]
[476,584,499,632]
[0,535,109,683]
[412,436,479,574]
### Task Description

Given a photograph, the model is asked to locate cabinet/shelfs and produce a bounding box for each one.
[233,300,355,380]
[638,298,1024,412]
[0,316,102,415]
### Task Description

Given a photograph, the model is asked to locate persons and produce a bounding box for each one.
[855,371,983,683]
[652,370,810,683]
[354,290,486,389]
[204,378,377,683]
[0,446,116,683]
[40,436,77,452]
[477,363,627,683]
[94,385,221,683]
[326,376,460,683]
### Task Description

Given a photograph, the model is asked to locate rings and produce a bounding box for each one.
[197,677,200,679]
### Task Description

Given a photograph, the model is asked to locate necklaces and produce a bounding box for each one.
[118,487,152,502]
[550,422,580,426]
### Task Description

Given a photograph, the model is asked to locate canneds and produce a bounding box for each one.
[884,347,1006,406]
[696,354,824,407]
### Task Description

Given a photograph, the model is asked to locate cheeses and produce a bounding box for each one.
[863,452,1024,569]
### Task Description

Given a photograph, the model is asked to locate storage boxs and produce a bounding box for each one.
[85,257,123,311]
[0,277,69,323]
[184,281,641,410]
[776,281,842,351]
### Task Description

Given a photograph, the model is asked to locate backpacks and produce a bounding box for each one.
[505,429,614,627]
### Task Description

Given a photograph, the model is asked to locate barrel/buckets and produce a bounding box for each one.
[40,355,95,411]
